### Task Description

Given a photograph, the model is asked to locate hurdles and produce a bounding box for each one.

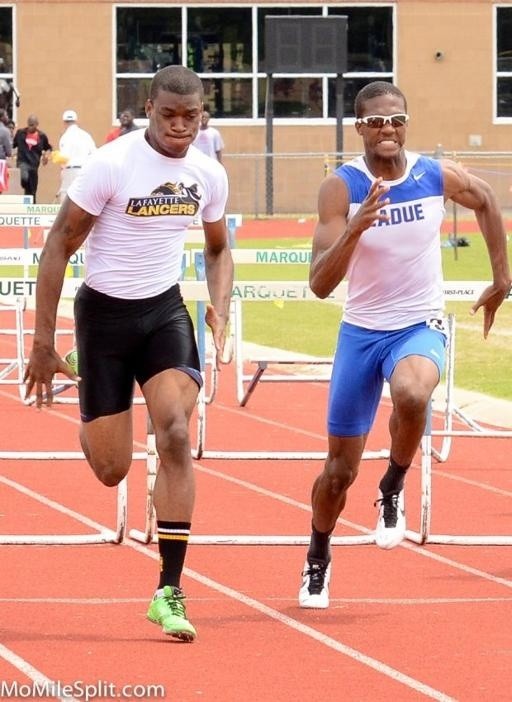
[0,195,512,545]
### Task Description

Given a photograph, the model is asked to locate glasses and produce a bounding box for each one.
[356,114,409,128]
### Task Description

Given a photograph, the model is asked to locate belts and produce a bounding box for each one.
[62,165,80,169]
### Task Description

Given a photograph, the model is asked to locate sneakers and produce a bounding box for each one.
[146,585,196,641]
[299,557,332,608]
[376,489,406,549]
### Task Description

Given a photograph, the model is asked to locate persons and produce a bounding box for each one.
[121,106,148,138]
[298,81,510,608]
[53,110,98,205]
[103,114,124,143]
[21,63,236,643]
[13,114,52,205]
[0,108,13,194]
[191,110,225,164]
[6,119,17,167]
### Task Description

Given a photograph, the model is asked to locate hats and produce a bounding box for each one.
[63,110,77,121]
